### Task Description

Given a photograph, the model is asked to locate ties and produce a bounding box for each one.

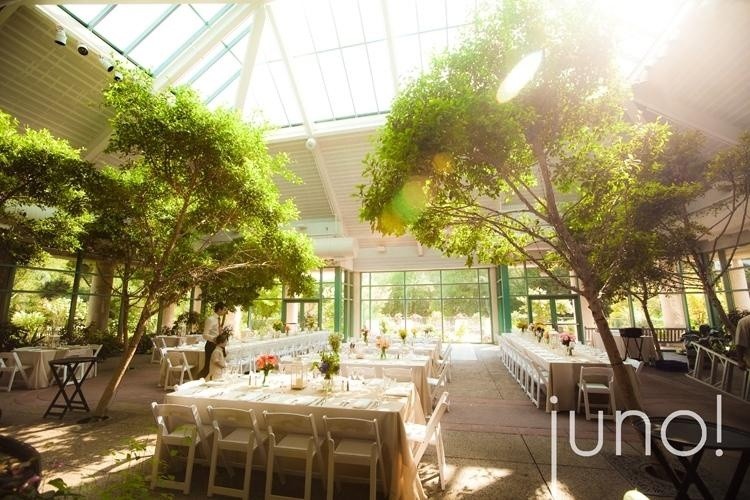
[218,317,221,335]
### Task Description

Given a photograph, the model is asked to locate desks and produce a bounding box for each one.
[41,357,98,420]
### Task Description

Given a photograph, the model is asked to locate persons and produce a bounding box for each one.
[699,323,723,347]
[734,314,750,371]
[203,335,228,382]
[192,301,227,381]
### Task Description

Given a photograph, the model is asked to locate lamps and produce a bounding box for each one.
[49,20,124,82]
[495,49,543,104]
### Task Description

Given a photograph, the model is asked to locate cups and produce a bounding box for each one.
[175,350,408,410]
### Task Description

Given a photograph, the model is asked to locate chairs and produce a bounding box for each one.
[151,329,452,500]
[1,351,33,392]
[497,329,646,421]
[87,344,104,377]
